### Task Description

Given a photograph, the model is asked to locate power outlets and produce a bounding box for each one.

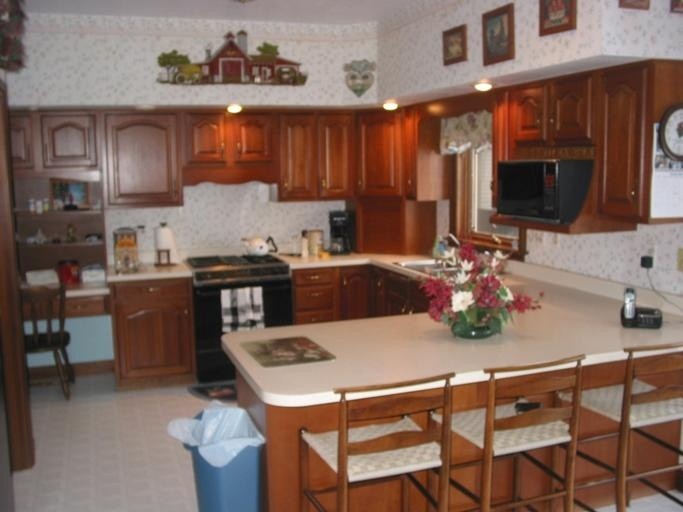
[640,252,652,267]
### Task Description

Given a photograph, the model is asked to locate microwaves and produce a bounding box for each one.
[494,157,597,227]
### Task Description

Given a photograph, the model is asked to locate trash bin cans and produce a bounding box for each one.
[167,400,267,512]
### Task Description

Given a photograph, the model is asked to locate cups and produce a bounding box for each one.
[127,251,139,276]
[112,251,127,276]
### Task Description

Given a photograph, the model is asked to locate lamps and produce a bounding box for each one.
[385,100,400,111]
[475,80,493,94]
[227,104,245,116]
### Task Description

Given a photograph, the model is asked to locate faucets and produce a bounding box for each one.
[439,233,460,249]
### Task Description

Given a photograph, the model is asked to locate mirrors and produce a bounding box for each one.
[455,113,523,256]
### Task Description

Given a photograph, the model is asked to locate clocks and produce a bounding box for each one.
[661,106,683,163]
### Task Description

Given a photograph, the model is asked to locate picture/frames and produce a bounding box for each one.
[539,0,576,35]
[669,0,683,15]
[48,177,93,210]
[443,23,468,64]
[618,0,652,12]
[482,3,516,65]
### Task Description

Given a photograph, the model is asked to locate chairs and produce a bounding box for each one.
[20,284,77,399]
[560,344,683,512]
[429,352,585,512]
[298,370,453,512]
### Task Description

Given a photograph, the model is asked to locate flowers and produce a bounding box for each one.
[419,242,545,329]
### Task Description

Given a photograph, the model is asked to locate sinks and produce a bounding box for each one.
[397,260,459,269]
[418,270,460,278]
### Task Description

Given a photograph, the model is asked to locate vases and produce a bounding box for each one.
[449,315,497,339]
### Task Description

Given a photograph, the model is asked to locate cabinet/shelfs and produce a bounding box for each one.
[42,113,98,175]
[108,262,194,392]
[374,253,433,315]
[356,109,417,204]
[269,111,357,199]
[288,252,374,321]
[496,79,638,233]
[594,58,683,222]
[179,112,280,186]
[12,110,37,172]
[222,291,683,512]
[104,111,181,206]
[17,175,109,316]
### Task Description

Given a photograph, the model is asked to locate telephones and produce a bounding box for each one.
[620,286,662,329]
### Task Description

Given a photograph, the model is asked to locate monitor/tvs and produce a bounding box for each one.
[497,159,561,225]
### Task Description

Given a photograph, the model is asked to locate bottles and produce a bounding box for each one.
[311,230,324,257]
[42,198,49,214]
[624,289,635,319]
[28,199,35,215]
[35,200,43,216]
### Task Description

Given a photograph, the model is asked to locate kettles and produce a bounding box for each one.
[239,237,279,257]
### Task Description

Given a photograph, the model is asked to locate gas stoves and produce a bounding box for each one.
[185,253,292,286]
